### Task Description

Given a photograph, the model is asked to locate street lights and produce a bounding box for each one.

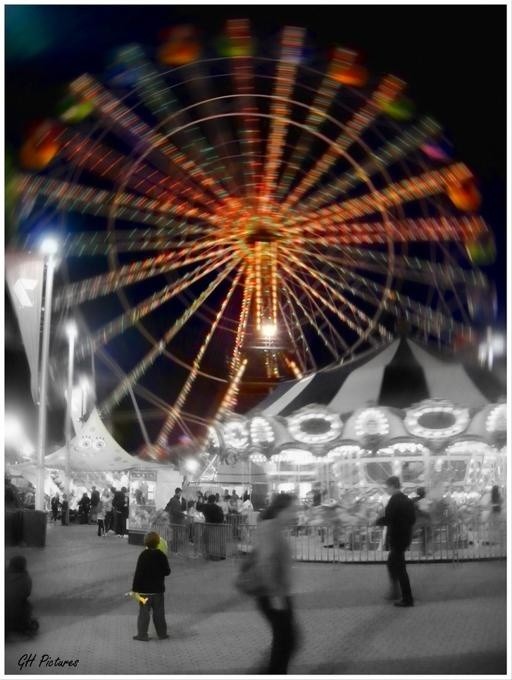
[31,233,63,522]
[58,318,83,528]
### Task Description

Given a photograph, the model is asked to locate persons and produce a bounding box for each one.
[407,482,505,557]
[5,477,36,511]
[130,531,170,641]
[371,475,419,610]
[246,493,305,674]
[6,554,38,635]
[46,487,254,561]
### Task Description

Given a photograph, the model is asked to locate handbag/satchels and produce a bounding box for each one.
[235,573,266,595]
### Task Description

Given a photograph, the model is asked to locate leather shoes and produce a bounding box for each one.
[394,599,414,607]
